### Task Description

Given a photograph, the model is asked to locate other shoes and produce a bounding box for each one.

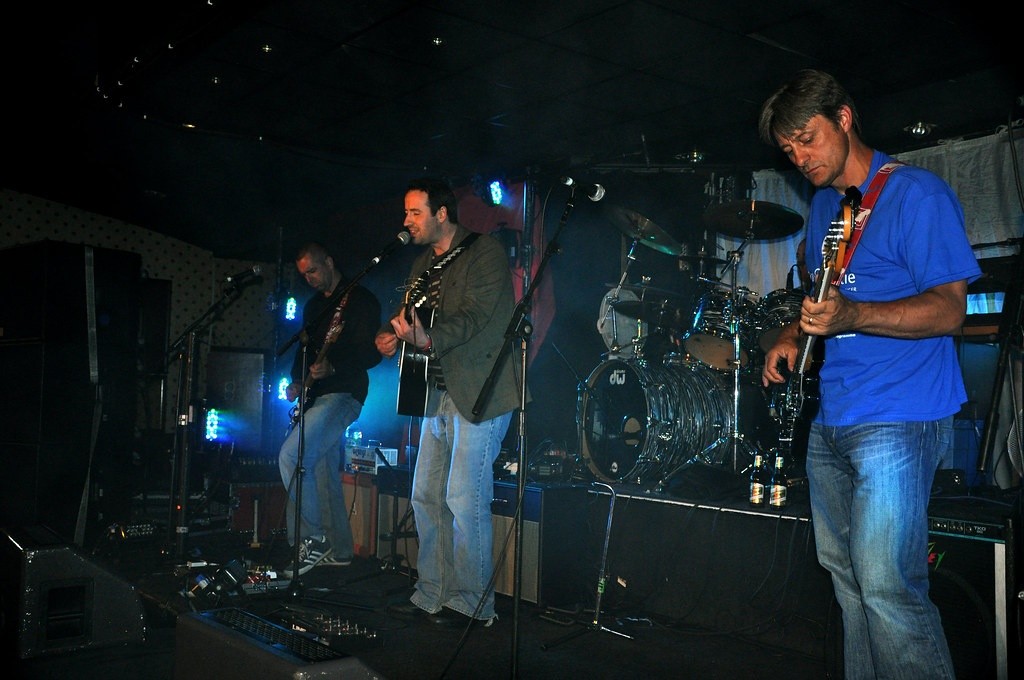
[393,601,453,618]
[429,609,494,629]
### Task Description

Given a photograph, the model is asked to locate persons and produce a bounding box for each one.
[757,68,983,680]
[374,175,533,629]
[278,239,383,578]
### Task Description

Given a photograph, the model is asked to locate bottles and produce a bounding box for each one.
[749,451,765,507]
[345,420,362,474]
[769,452,788,510]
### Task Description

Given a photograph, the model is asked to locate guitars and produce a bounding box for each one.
[396,246,466,417]
[769,204,854,455]
[289,285,354,429]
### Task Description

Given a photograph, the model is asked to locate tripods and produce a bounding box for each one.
[248,336,391,612]
[325,483,418,589]
[539,482,636,649]
[652,216,779,494]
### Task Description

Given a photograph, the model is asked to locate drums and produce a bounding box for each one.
[577,351,734,486]
[754,288,808,354]
[682,289,756,372]
[598,288,649,358]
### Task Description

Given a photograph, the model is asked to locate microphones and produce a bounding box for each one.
[607,432,639,441]
[639,130,651,170]
[371,231,410,265]
[225,265,264,284]
[560,176,606,202]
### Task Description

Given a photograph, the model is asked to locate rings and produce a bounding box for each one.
[808,317,813,324]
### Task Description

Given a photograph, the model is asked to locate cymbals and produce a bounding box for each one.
[595,204,682,256]
[705,200,804,240]
[605,282,685,299]
[678,256,729,265]
[611,302,676,327]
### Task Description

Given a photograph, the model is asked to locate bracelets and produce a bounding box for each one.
[420,334,432,353]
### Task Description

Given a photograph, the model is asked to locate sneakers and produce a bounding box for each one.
[317,551,350,567]
[284,534,333,577]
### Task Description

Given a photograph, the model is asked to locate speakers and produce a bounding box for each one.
[1,240,142,526]
[0,525,146,659]
[173,610,388,680]
